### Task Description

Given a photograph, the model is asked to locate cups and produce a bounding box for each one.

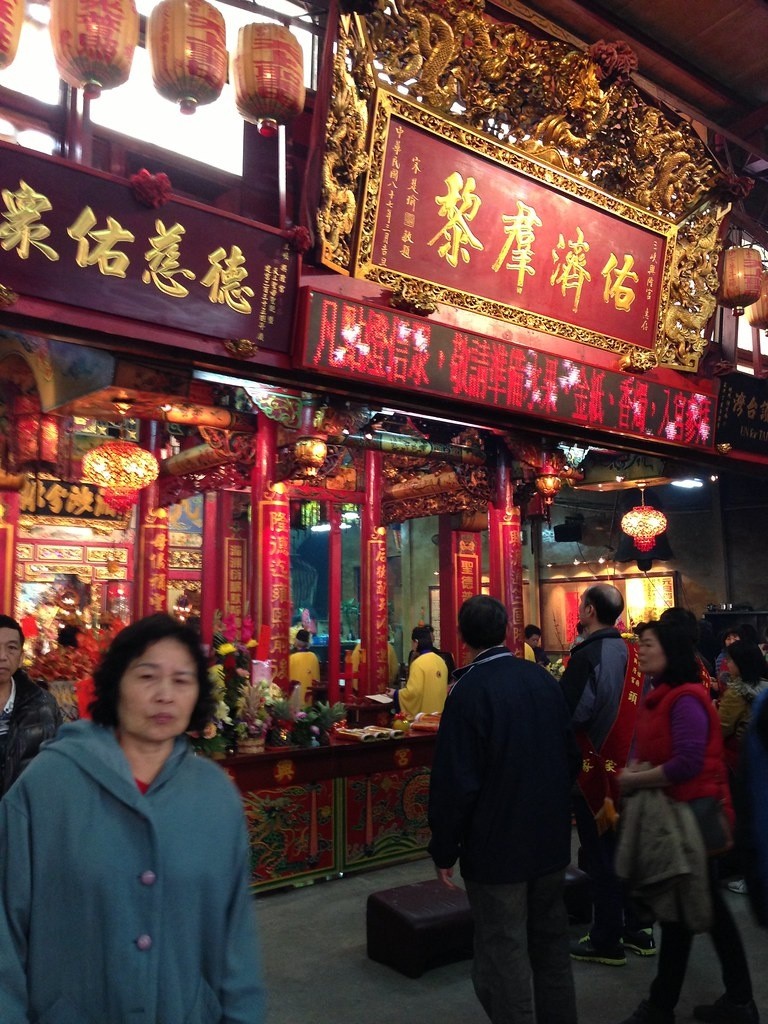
[721,603,733,611]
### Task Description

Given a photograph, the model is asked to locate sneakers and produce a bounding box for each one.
[569,932,628,966]
[618,927,658,956]
[693,993,760,1024]
[619,999,676,1024]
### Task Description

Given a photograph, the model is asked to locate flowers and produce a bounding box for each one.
[186,644,281,754]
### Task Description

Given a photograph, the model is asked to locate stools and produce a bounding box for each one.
[366,876,472,979]
[563,866,593,925]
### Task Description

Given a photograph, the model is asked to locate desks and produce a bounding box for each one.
[209,729,442,897]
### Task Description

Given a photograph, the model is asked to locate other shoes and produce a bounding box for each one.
[728,878,750,896]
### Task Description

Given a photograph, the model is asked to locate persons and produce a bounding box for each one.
[525,624,554,668]
[611,604,768,1024]
[288,629,320,713]
[569,623,589,660]
[382,624,457,721]
[424,596,579,1024]
[1,611,267,1024]
[0,614,65,801]
[558,584,658,966]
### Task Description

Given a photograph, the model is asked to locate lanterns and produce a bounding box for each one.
[49,1,141,99]
[717,248,761,316]
[81,439,159,517]
[0,0,26,70]
[232,23,305,136]
[619,504,668,553]
[747,270,768,337]
[145,0,227,116]
